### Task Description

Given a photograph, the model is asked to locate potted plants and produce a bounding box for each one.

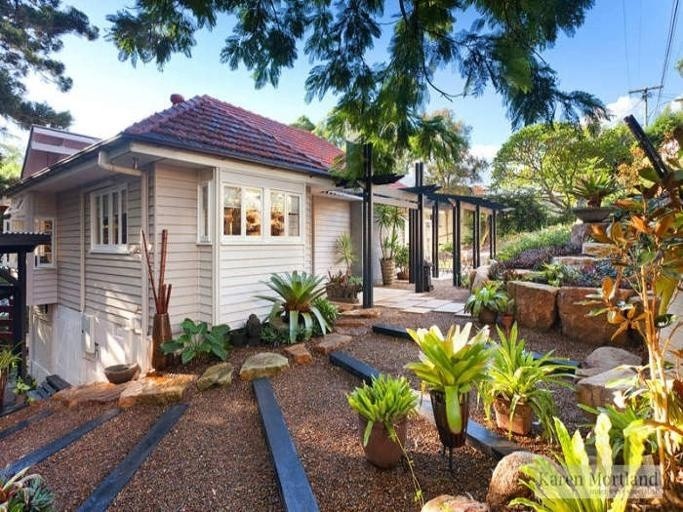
[0,341,22,412]
[406,318,493,448]
[565,174,628,221]
[12,380,29,407]
[478,333,582,435]
[342,373,417,466]
[395,245,409,279]
[374,207,403,284]
[493,295,512,327]
[231,330,246,346]
[464,275,499,326]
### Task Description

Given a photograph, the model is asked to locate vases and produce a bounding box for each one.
[105,363,139,384]
[151,313,171,371]
